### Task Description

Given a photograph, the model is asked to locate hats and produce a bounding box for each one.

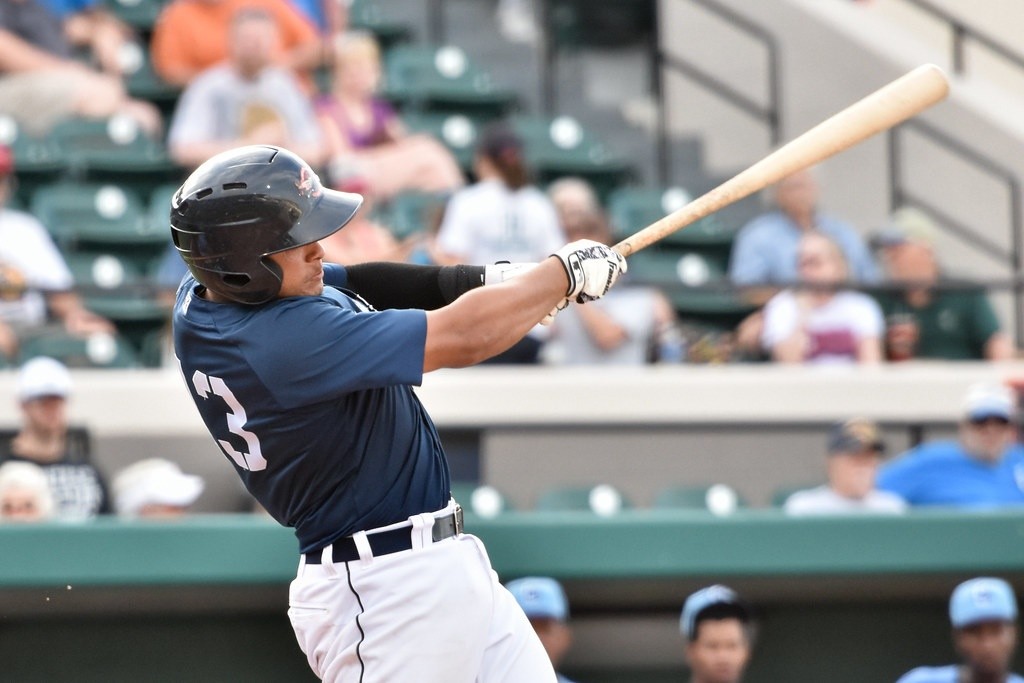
[16,356,67,403]
[678,587,742,641]
[827,424,886,455]
[965,385,1011,422]
[948,578,1019,626]
[865,209,938,254]
[507,575,568,623]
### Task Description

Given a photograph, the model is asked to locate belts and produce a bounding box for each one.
[305,508,464,567]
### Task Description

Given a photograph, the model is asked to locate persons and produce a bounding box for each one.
[0,0,163,139]
[170,145,628,683]
[0,144,119,363]
[779,415,911,514]
[149,0,466,267]
[1,356,205,522]
[895,576,1024,683]
[677,584,754,683]
[431,122,686,365]
[727,165,1018,365]
[504,576,573,682]
[872,381,1024,507]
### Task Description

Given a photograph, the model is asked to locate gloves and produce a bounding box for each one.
[553,238,627,306]
[486,262,564,326]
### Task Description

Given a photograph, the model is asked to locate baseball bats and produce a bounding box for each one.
[539,63,951,327]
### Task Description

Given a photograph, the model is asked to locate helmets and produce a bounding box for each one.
[169,143,363,306]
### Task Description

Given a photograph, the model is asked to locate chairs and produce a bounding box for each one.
[1,43,792,519]
